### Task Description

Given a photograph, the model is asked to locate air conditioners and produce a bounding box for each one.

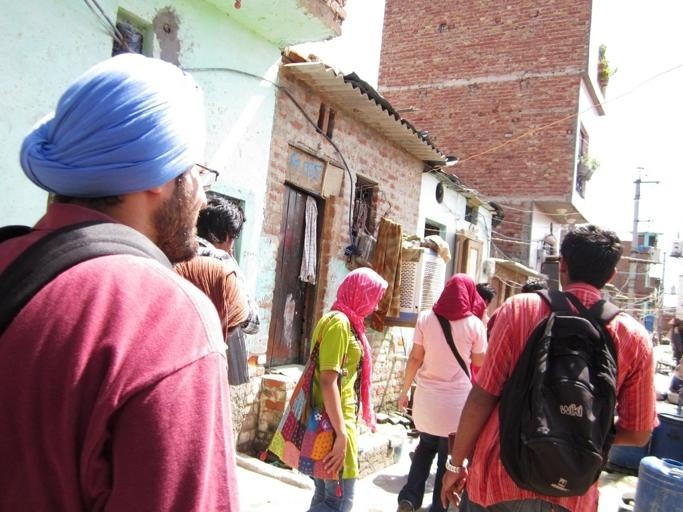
[400,246,448,314]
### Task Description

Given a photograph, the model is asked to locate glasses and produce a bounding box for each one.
[194,163,219,191]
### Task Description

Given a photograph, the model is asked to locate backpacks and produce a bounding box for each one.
[499,288,625,497]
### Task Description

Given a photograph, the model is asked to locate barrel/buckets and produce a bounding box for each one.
[608,407,649,470]
[632,455,683,512]
[649,412,683,463]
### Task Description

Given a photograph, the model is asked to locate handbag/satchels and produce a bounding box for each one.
[268,311,348,481]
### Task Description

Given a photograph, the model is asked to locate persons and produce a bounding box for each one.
[0,53,238,512]
[396,274,487,512]
[477,283,496,325]
[175,197,261,386]
[442,226,658,512]
[304,268,389,512]
[488,280,549,342]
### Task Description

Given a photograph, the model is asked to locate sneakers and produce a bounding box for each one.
[397,499,415,512]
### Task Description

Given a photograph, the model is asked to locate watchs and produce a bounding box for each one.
[446,454,464,473]
